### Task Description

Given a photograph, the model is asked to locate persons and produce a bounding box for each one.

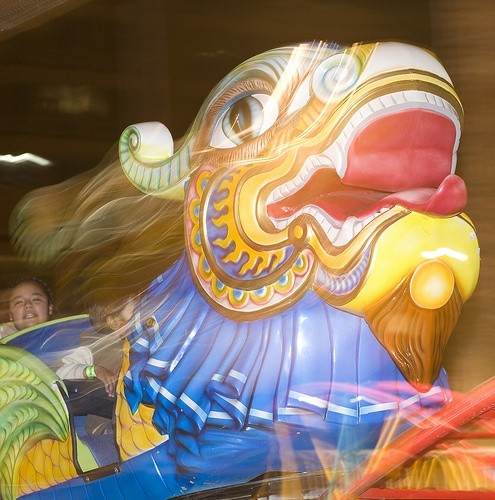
[54,284,145,467]
[0,275,55,343]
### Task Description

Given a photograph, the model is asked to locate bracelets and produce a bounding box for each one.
[84,365,98,379]
